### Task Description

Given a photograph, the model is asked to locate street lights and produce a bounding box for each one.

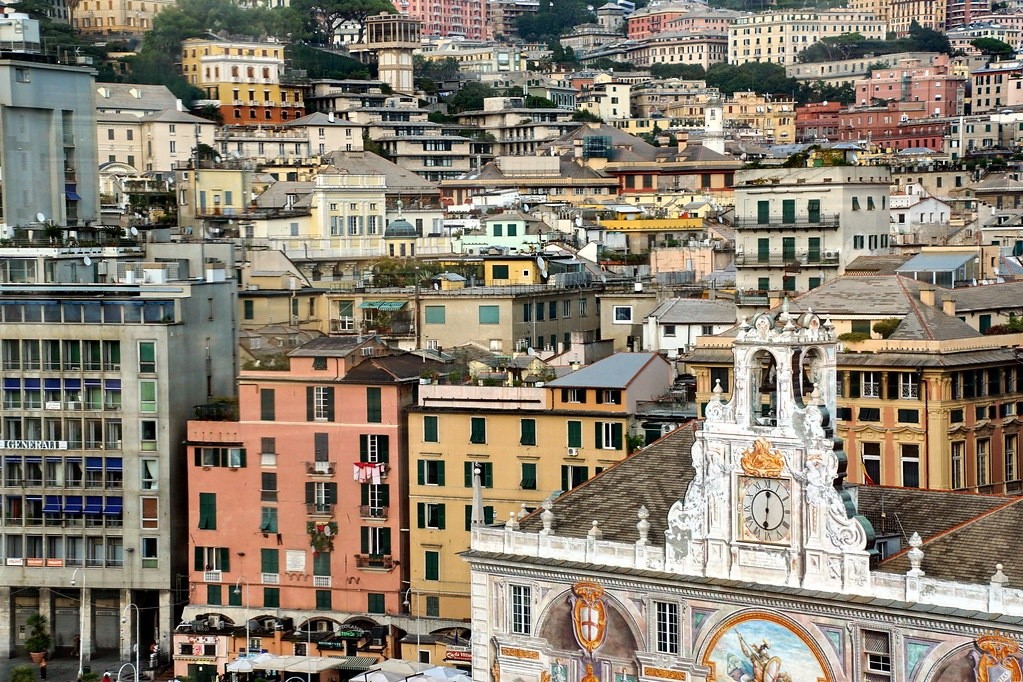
[233,576,251,682]
[402,586,422,663]
[120,603,140,682]
[293,615,311,682]
[69,568,85,678]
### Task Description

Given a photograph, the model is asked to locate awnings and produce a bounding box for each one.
[327,655,380,670]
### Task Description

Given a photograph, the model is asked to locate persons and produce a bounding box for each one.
[101,669,117,682]
[40,659,47,680]
[211,672,227,682]
[132,642,137,661]
[150,643,156,654]
[70,634,80,656]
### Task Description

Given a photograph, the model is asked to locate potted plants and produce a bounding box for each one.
[534,367,545,387]
[23,610,52,663]
[419,365,433,385]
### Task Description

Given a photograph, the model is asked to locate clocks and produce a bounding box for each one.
[739,474,792,544]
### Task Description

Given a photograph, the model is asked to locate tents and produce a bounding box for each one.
[349,658,472,682]
[227,652,348,673]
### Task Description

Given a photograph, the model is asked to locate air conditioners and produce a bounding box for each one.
[207,615,221,627]
[568,448,578,455]
[317,620,329,631]
[265,619,277,629]
[372,638,382,646]
